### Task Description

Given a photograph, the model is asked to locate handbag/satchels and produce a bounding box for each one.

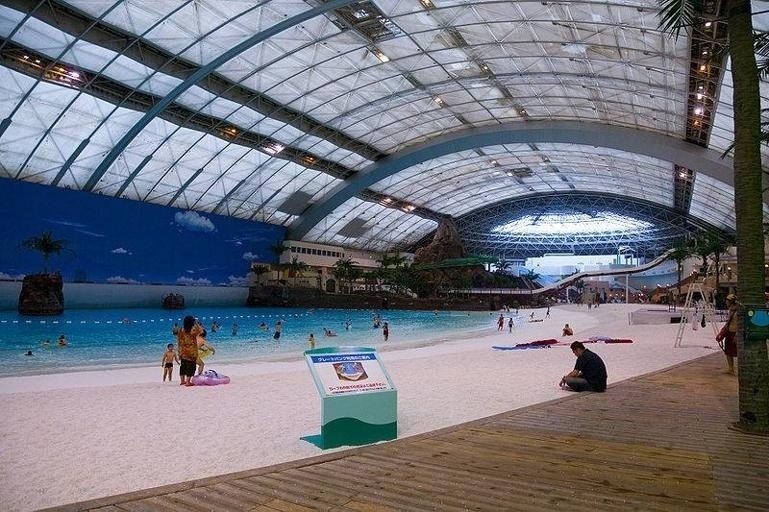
[716,324,729,342]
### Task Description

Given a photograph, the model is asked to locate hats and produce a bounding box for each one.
[726,294,737,300]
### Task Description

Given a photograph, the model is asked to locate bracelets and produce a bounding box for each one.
[562,377,566,382]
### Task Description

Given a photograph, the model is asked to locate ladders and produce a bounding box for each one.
[674,283,724,348]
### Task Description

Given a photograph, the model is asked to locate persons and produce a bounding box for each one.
[562,323,573,336]
[194,328,215,374]
[720,292,739,377]
[497,313,504,331]
[161,344,180,382]
[25,334,69,358]
[507,317,514,332]
[176,315,205,386]
[559,340,607,393]
[172,310,408,357]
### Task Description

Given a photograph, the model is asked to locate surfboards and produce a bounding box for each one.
[493,345,547,350]
[198,346,215,362]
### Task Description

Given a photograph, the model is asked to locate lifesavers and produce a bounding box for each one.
[191,370,230,386]
